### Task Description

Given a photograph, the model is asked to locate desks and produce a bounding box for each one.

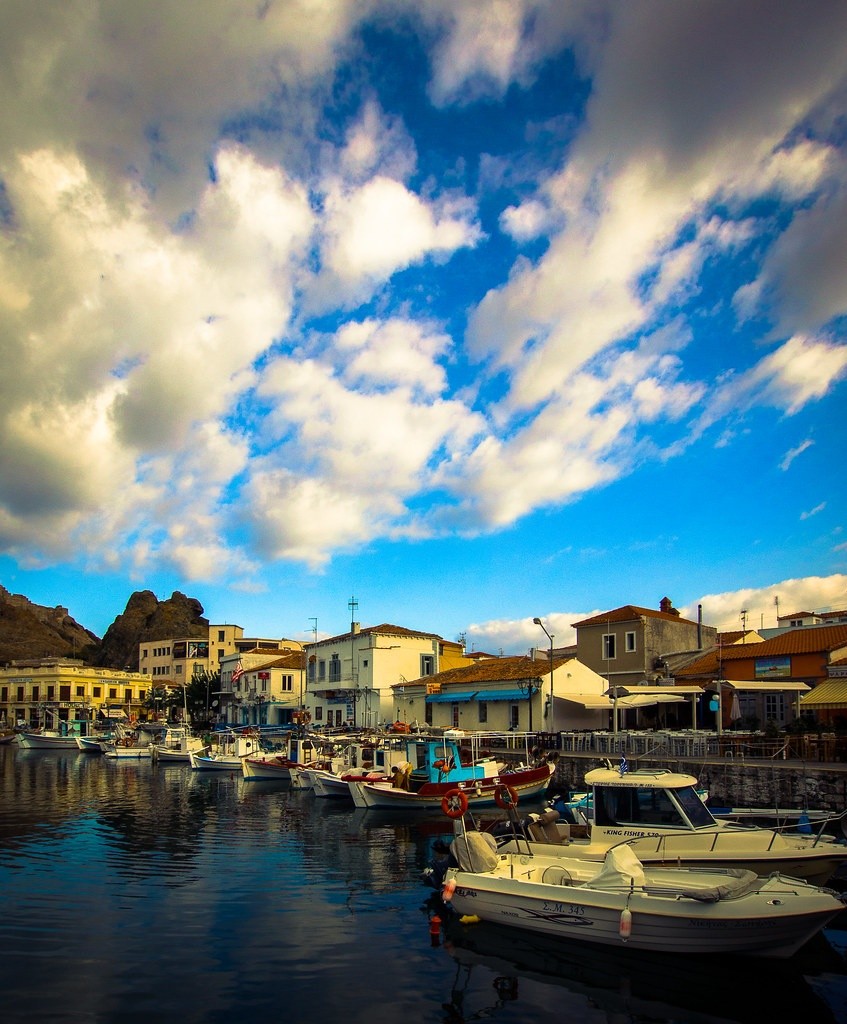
[504,729,847,764]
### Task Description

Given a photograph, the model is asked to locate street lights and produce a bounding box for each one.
[533,617,555,732]
[282,638,303,711]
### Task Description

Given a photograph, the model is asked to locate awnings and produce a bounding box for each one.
[474,688,537,700]
[703,680,811,690]
[425,691,479,702]
[101,709,128,718]
[553,685,705,709]
[792,678,847,709]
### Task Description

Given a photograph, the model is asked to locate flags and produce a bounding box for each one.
[620,753,628,776]
[231,661,245,683]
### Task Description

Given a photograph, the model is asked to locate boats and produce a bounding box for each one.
[563,788,841,824]
[1,685,555,810]
[495,767,846,888]
[440,828,847,952]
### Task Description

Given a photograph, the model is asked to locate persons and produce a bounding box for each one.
[325,720,353,737]
[392,761,413,792]
[202,732,215,756]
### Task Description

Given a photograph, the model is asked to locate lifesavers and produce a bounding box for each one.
[300,710,312,725]
[116,739,123,746]
[394,721,410,731]
[123,738,133,747]
[460,747,488,767]
[494,784,519,811]
[441,789,468,818]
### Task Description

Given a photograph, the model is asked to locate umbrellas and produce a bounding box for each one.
[730,694,742,731]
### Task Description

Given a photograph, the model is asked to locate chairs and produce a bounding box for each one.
[606,736,621,753]
[810,734,818,750]
[582,733,591,752]
[510,733,524,749]
[660,734,668,755]
[616,736,627,753]
[773,735,791,760]
[569,733,583,752]
[699,736,707,756]
[830,733,836,754]
[629,733,644,753]
[818,733,830,762]
[649,734,661,755]
[684,737,700,757]
